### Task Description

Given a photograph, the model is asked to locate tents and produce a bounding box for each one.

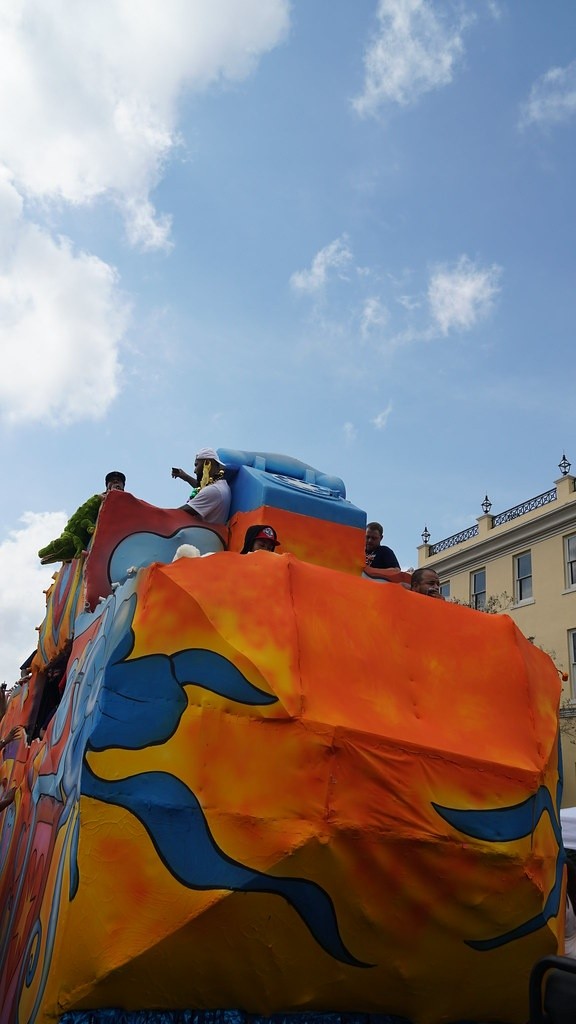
[559,807,576,852]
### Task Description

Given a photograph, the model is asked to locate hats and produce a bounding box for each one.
[106,471,125,487]
[253,527,281,546]
[196,448,226,467]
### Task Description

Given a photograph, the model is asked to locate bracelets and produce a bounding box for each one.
[2,740,6,744]
[2,800,7,807]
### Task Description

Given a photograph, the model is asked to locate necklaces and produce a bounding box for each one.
[209,476,223,485]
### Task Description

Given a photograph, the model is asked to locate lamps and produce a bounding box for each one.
[557,449,572,476]
[481,490,492,515]
[420,527,431,544]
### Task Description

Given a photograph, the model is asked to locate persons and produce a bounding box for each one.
[172,449,232,525]
[410,568,446,601]
[239,524,281,557]
[33,652,69,740]
[0,728,19,812]
[364,522,401,571]
[563,852,576,960]
[99,472,126,500]
